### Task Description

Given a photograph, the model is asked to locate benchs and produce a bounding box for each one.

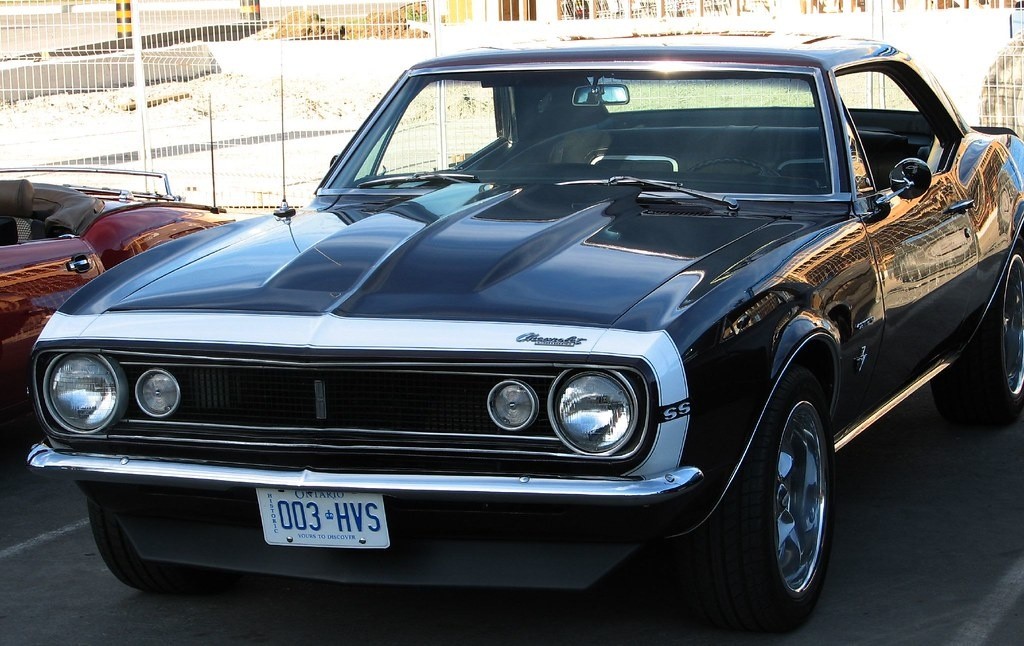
[514,123,910,191]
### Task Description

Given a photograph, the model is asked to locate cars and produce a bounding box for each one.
[25,40,1023,640]
[0,169,261,438]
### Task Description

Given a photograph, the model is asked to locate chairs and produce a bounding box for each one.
[0,179,46,243]
